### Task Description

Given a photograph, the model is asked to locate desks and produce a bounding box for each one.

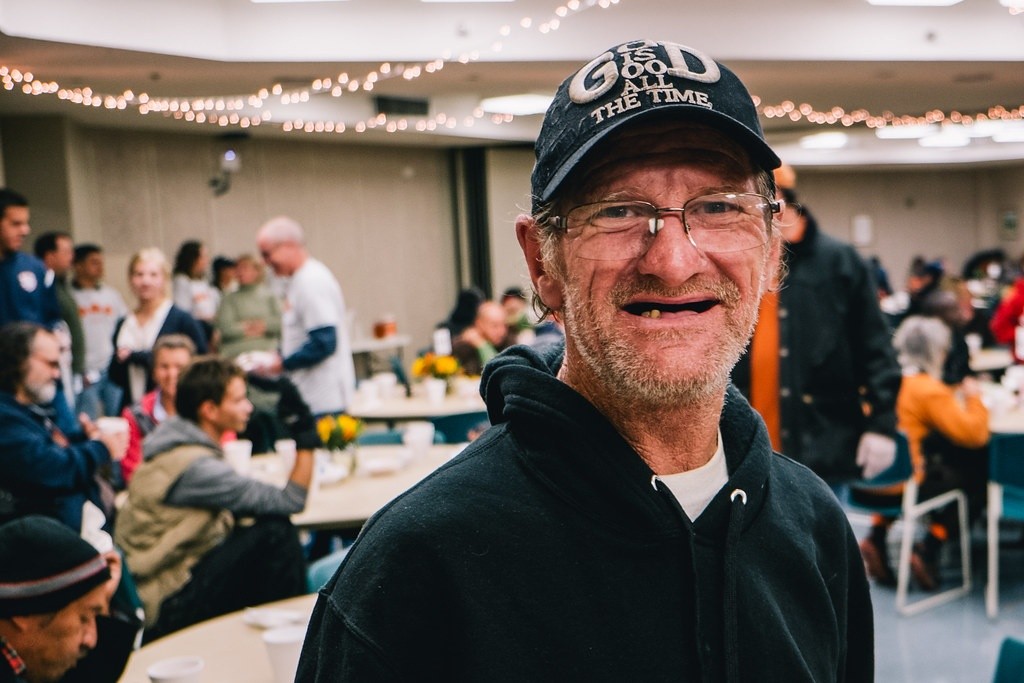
[114,442,469,566]
[116,592,319,683]
[349,334,410,380]
[345,376,489,445]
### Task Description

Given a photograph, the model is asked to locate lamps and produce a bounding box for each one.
[209,150,243,197]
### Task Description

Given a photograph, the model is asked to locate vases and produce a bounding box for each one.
[326,446,352,478]
[439,377,455,397]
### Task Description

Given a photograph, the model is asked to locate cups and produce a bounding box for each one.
[263,621,307,683]
[220,377,480,476]
[148,657,204,683]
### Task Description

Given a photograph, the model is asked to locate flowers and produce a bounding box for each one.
[316,415,368,452]
[411,353,465,385]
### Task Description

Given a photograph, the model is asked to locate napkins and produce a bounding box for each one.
[243,605,308,628]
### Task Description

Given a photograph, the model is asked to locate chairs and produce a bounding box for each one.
[984,433,1024,619]
[836,429,973,616]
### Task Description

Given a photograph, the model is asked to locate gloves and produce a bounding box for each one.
[276,393,321,449]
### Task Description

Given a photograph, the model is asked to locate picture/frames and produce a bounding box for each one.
[997,204,1022,241]
[849,211,878,247]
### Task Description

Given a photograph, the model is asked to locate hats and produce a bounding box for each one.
[0,516,113,620]
[529,40,782,226]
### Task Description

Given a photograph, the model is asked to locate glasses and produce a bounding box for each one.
[547,192,780,260]
[30,353,59,369]
[260,240,283,257]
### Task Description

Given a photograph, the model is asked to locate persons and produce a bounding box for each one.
[0,184,358,682]
[435,281,566,375]
[291,37,879,683]
[853,247,1024,594]
[728,162,906,513]
